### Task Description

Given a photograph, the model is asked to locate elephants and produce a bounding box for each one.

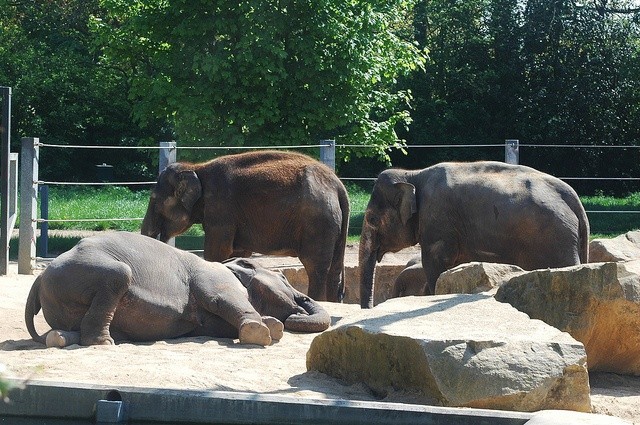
[360,160,590,309]
[141,150,350,302]
[25,231,331,348]
